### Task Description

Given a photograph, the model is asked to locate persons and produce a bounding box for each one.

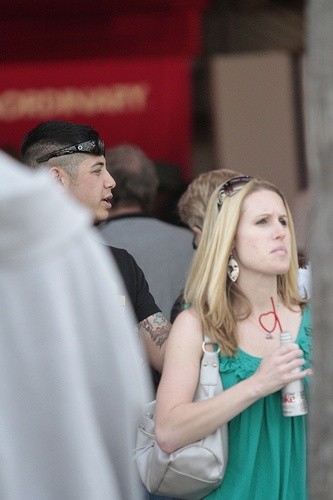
[155,175,314,500]
[0,118,316,500]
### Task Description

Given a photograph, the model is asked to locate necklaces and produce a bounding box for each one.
[248,298,280,339]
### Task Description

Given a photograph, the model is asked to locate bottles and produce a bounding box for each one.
[279,331,308,417]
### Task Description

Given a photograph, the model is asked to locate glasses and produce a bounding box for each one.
[217,176,255,212]
[35,139,105,163]
[192,233,198,250]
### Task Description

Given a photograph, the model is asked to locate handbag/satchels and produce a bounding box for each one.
[136,337,228,500]
